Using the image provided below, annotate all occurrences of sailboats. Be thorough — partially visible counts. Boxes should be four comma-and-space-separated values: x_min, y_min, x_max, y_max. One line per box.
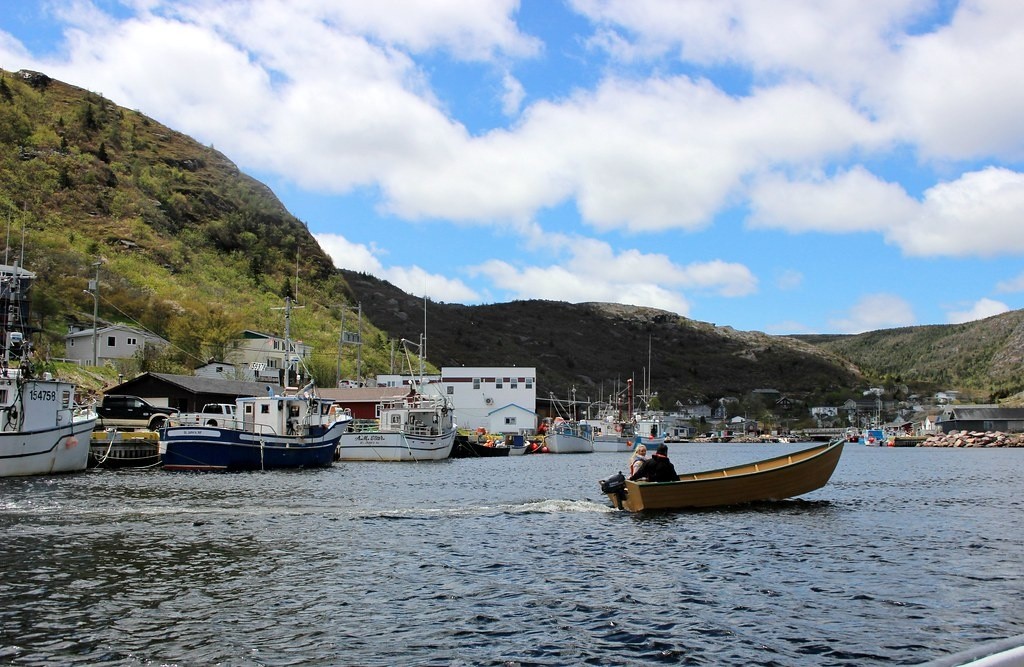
544, 332, 668, 453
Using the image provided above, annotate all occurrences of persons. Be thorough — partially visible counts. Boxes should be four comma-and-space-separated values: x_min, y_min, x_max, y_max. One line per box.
627, 443, 681, 482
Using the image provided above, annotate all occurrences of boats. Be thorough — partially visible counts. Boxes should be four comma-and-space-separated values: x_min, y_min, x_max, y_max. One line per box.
0, 201, 459, 478
845, 389, 887, 446
499, 440, 530, 456
466, 440, 511, 456
599, 437, 846, 513
